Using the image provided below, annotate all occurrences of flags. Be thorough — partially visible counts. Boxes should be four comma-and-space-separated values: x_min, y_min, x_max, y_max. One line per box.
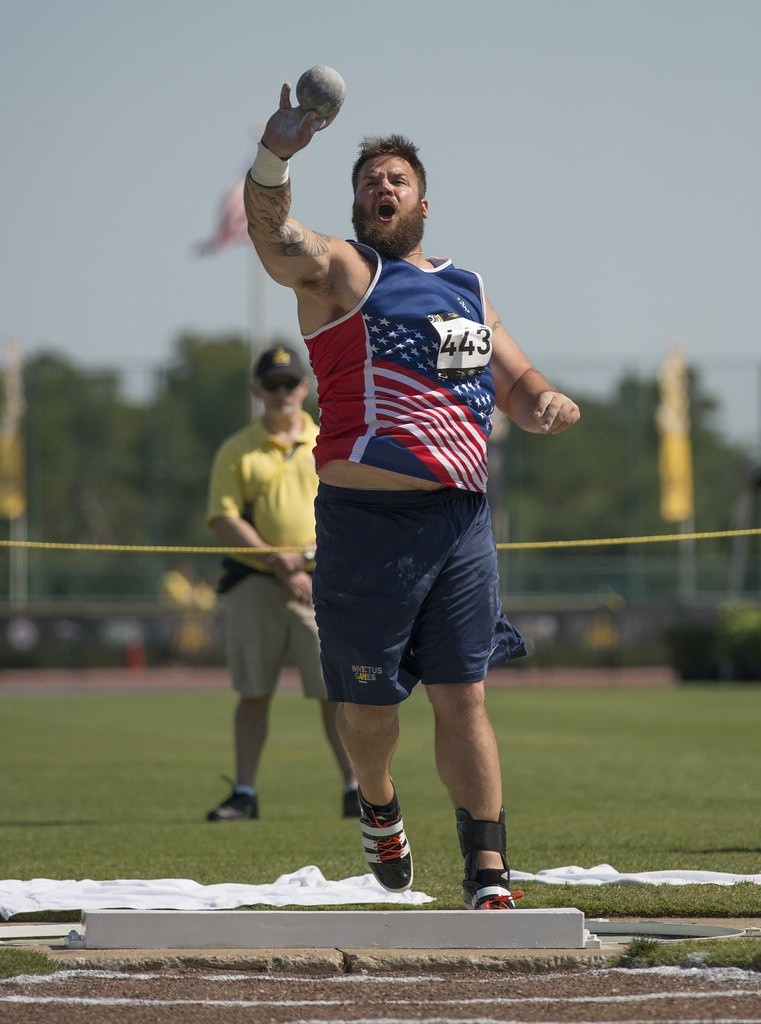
192, 181, 256, 255
0, 358, 30, 520
652, 344, 694, 523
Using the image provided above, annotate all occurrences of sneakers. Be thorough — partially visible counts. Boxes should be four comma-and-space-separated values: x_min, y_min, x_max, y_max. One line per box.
356, 775, 415, 894
343, 788, 362, 819
205, 773, 260, 823
460, 877, 525, 910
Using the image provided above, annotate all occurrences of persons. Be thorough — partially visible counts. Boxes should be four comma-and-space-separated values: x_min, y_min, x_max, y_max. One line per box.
245, 82, 579, 911
163, 346, 358, 824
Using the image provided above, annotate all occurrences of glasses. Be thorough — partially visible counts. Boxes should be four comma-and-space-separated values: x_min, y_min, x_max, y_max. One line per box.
264, 376, 300, 392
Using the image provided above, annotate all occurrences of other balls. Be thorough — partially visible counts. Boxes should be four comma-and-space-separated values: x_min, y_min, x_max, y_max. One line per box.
293, 64, 351, 119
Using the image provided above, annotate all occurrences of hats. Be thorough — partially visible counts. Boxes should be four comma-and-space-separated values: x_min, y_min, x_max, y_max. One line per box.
253, 342, 307, 387
